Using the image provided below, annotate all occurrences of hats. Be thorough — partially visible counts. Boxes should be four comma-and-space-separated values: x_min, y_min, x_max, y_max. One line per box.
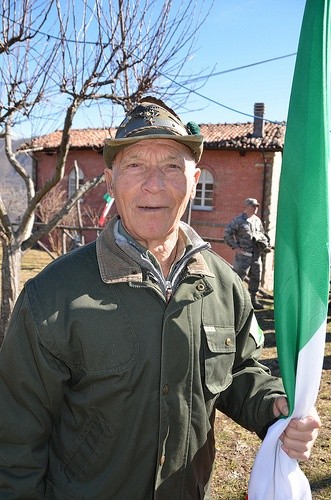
244, 198, 260, 207
102, 101, 204, 168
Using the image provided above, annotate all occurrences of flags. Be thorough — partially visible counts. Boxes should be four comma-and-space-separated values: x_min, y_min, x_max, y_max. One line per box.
246, 0, 327, 500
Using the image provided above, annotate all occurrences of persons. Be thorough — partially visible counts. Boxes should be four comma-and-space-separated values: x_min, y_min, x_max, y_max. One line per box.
221, 197, 273, 311
0, 97, 323, 500
